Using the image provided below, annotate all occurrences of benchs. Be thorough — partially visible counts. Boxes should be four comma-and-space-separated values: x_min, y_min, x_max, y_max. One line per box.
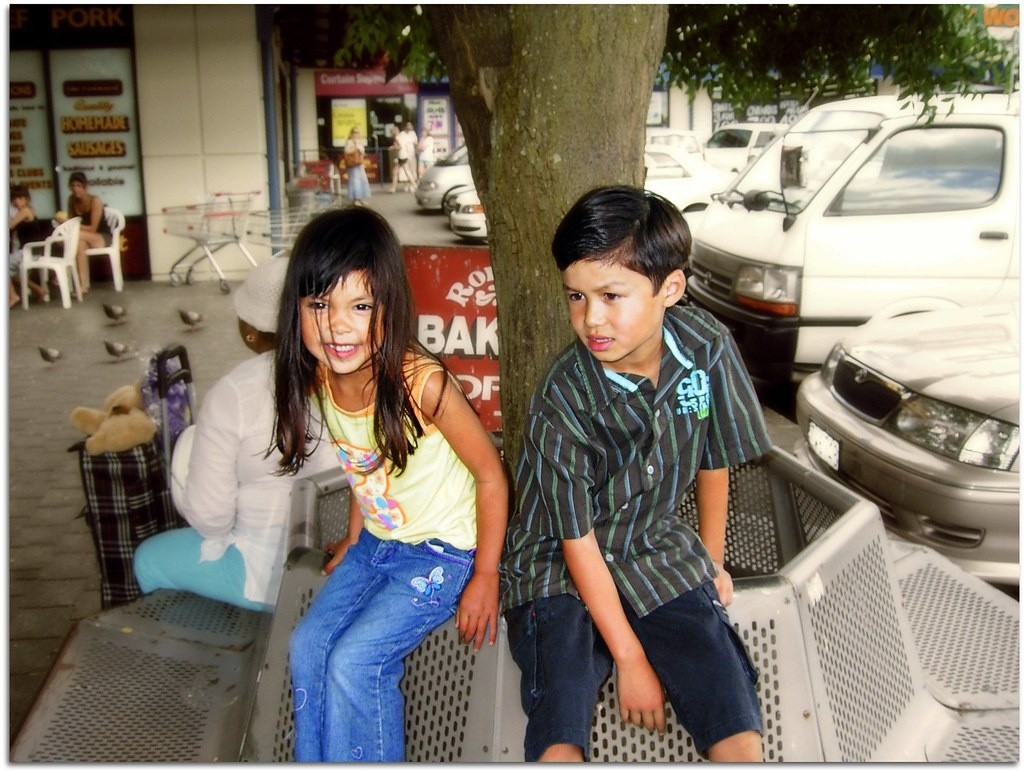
10, 449, 1019, 763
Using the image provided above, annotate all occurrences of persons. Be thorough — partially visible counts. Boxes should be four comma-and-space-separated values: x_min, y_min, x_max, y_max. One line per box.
264, 206, 509, 763
9, 184, 51, 310
387, 121, 435, 195
135, 256, 339, 612
344, 127, 372, 206
499, 184, 769, 763
53, 173, 114, 299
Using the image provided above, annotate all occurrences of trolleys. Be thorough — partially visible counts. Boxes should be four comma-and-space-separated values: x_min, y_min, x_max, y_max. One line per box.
246, 189, 357, 259
162, 191, 262, 294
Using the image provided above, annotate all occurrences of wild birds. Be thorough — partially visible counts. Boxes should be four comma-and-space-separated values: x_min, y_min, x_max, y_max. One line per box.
98, 301, 129, 326
173, 306, 206, 331
102, 339, 130, 362
36, 345, 67, 366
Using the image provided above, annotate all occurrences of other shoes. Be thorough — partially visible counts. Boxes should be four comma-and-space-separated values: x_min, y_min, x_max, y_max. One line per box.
38, 292, 50, 302
349, 200, 369, 208
47, 279, 61, 289
7, 296, 21, 309
70, 288, 90, 299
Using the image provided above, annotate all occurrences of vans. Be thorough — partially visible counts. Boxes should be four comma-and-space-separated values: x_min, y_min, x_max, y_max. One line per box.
690, 93, 1022, 397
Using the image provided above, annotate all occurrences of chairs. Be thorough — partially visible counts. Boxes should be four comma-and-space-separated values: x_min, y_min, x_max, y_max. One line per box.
79, 208, 127, 292
20, 217, 81, 311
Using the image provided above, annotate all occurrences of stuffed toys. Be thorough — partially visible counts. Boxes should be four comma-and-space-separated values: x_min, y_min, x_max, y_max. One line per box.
72, 384, 156, 456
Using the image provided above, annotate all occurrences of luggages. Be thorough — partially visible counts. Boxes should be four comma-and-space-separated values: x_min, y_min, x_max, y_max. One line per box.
69, 341, 197, 608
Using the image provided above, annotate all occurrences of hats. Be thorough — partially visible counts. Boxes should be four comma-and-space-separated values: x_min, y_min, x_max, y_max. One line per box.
69, 172, 87, 183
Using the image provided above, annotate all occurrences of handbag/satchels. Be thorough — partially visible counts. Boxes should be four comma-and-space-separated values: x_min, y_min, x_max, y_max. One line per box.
344, 149, 365, 169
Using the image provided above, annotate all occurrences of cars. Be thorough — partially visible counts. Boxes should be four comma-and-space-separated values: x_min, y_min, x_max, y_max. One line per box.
451, 143, 740, 247
794, 291, 1020, 585
699, 121, 790, 175
415, 125, 700, 217
